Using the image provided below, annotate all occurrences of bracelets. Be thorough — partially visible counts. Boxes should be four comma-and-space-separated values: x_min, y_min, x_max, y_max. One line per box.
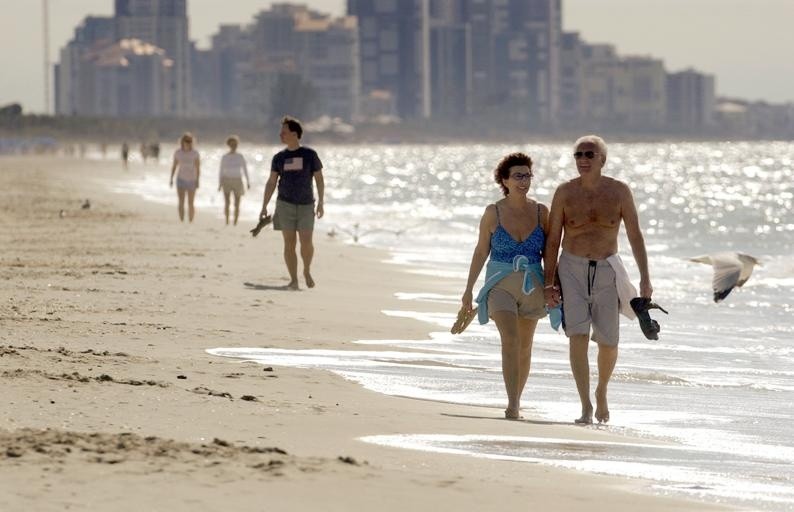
543, 285, 553, 289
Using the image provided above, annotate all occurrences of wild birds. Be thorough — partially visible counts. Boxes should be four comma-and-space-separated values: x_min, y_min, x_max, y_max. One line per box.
684, 252, 763, 304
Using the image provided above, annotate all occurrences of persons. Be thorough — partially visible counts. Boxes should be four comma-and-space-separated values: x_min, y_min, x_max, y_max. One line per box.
169, 132, 200, 223
259, 115, 325, 290
461, 152, 560, 418
543, 135, 652, 424
217, 135, 251, 226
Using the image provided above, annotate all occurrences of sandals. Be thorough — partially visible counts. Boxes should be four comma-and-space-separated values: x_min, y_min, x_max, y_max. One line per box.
629, 296, 661, 341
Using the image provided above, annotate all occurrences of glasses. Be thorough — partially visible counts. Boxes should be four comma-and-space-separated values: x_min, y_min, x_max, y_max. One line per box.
573, 152, 600, 160
509, 172, 531, 181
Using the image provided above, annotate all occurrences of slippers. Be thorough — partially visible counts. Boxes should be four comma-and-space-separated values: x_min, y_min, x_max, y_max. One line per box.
451, 304, 479, 334
250, 215, 271, 238
